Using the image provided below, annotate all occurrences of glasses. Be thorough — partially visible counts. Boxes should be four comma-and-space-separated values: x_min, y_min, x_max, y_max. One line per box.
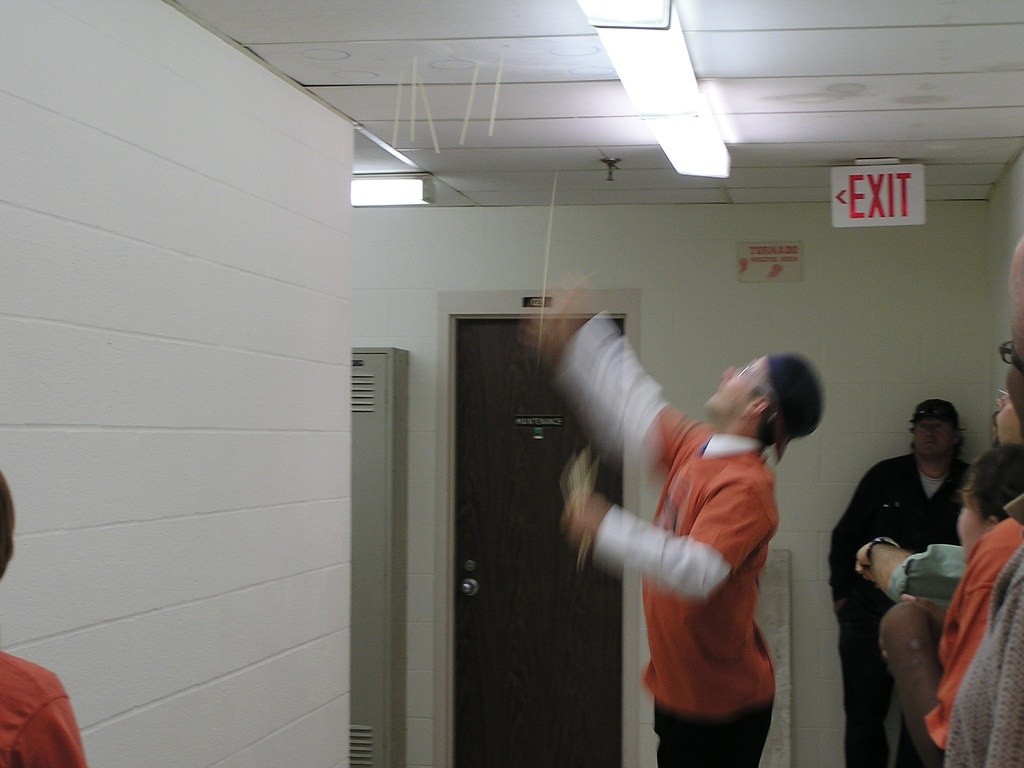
997, 388, 1010, 400
737, 363, 774, 397
1000, 339, 1017, 364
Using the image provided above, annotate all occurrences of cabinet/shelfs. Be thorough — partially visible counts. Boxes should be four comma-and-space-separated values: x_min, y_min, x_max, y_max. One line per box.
349, 347, 409, 768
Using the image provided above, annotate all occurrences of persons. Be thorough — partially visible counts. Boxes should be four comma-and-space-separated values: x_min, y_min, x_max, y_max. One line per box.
0, 469, 88, 768
556, 309, 825, 768
829, 233, 1023, 767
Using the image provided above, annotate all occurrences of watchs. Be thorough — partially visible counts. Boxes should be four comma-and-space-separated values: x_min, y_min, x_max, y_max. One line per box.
866, 537, 898, 564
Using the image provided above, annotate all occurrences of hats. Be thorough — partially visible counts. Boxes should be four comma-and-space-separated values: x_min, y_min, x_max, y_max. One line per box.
909, 399, 960, 430
768, 352, 822, 463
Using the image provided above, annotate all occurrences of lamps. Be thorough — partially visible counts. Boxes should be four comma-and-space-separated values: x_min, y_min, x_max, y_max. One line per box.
578, 0, 730, 178
351, 173, 436, 207
